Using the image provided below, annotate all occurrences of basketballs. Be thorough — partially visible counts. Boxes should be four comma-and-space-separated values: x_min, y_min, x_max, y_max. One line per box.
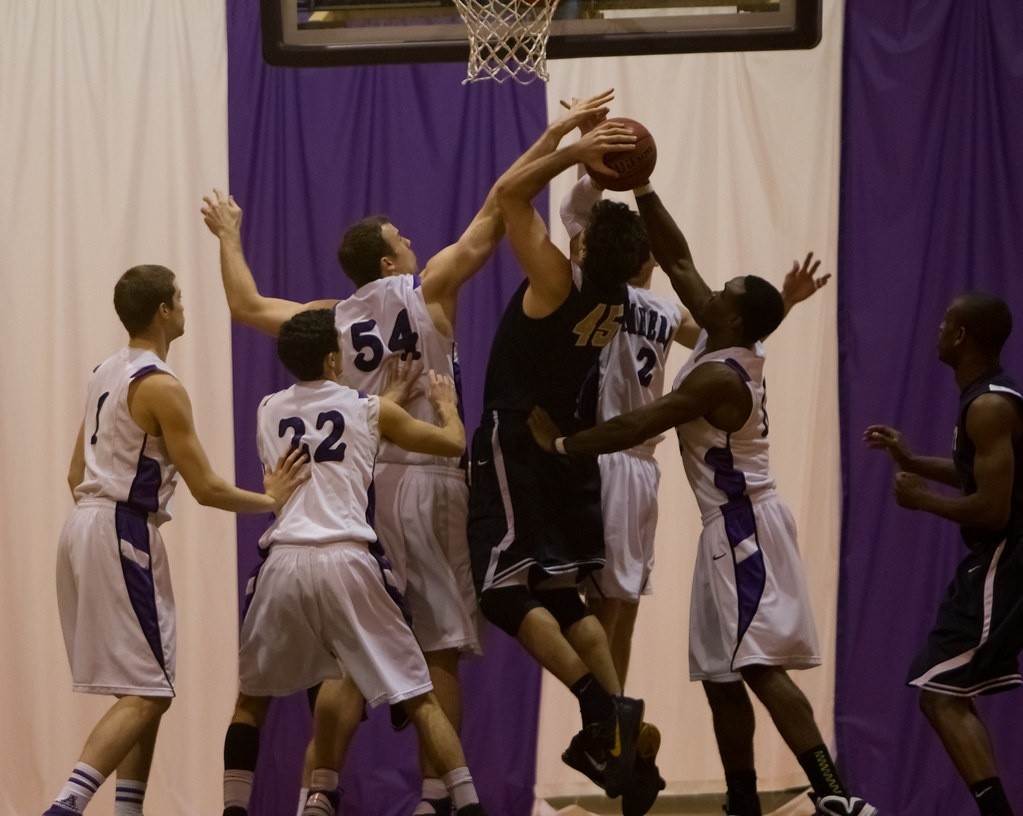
585, 117, 658, 192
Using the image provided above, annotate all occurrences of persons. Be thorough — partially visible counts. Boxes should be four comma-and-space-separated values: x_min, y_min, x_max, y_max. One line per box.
199, 88, 878, 816
862, 293, 1023, 816
41, 265, 313, 816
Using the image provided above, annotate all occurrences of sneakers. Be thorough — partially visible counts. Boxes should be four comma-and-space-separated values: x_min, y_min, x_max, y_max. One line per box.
412, 795, 458, 816
302, 787, 340, 816
561, 696, 666, 816
806, 790, 879, 816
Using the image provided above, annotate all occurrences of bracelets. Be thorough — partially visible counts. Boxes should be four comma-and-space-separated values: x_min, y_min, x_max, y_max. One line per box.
554, 436, 568, 455
633, 182, 654, 197
